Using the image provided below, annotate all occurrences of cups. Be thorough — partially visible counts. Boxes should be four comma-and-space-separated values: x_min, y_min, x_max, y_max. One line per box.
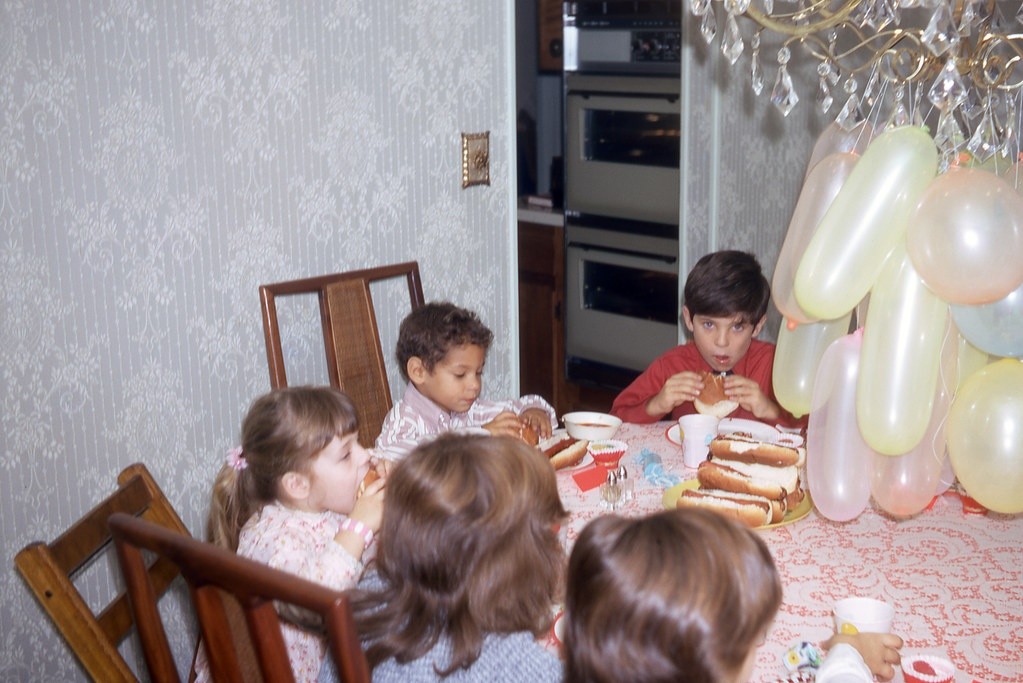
677, 413, 720, 468
831, 596, 895, 636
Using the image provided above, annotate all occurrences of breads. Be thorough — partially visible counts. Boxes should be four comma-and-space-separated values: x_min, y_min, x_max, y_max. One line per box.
358, 466, 386, 498
692, 370, 740, 418
508, 417, 540, 447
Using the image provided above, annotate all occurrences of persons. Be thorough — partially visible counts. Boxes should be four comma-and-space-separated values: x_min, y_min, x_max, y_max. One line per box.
193, 388, 396, 683
317, 434, 564, 683
611, 250, 809, 432
375, 303, 558, 447
562, 505, 903, 683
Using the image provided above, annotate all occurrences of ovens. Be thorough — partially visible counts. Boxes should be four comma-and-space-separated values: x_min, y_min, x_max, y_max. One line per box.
563, 69, 681, 373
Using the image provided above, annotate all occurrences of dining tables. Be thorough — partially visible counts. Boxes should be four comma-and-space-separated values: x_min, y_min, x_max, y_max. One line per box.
530, 418, 1023, 683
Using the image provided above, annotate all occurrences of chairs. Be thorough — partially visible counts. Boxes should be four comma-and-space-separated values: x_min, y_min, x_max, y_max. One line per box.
14, 461, 203, 683
107, 511, 373, 683
258, 261, 428, 449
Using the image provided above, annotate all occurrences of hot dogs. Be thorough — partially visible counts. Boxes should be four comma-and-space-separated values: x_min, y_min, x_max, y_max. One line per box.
676, 430, 804, 526
532, 433, 591, 470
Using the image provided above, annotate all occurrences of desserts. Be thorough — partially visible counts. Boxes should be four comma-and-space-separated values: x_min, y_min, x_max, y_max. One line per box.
909, 659, 938, 677
591, 443, 618, 453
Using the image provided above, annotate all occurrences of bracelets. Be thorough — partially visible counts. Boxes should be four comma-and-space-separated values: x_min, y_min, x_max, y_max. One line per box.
339, 518, 373, 550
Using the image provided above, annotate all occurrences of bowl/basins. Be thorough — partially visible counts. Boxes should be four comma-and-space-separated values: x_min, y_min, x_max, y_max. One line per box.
563, 411, 623, 441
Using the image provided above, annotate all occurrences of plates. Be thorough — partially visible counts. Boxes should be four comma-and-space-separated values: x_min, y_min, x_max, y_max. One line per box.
661, 478, 814, 530
665, 418, 783, 449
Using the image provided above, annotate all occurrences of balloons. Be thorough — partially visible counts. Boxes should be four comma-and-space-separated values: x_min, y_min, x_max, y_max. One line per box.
772, 121, 1023, 522
